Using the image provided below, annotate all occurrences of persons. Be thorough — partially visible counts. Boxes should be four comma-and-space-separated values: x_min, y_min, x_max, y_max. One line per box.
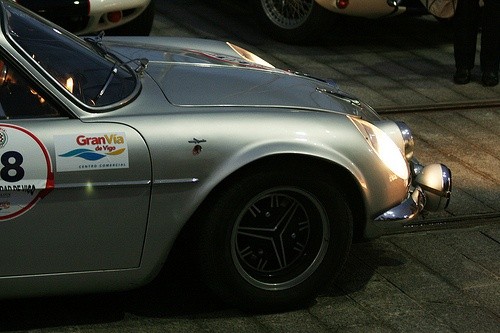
452, 0, 500, 87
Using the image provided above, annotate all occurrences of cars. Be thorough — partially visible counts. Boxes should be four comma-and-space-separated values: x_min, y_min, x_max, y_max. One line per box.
0, 0, 452, 314
9, 0, 156, 36
237, 0, 457, 44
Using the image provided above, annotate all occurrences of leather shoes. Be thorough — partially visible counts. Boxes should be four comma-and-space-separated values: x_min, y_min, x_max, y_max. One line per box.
482, 71, 498, 87
454, 68, 470, 84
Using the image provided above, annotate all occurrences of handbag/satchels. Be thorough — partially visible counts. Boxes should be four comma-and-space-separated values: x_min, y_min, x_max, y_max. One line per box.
421, 0, 457, 18
